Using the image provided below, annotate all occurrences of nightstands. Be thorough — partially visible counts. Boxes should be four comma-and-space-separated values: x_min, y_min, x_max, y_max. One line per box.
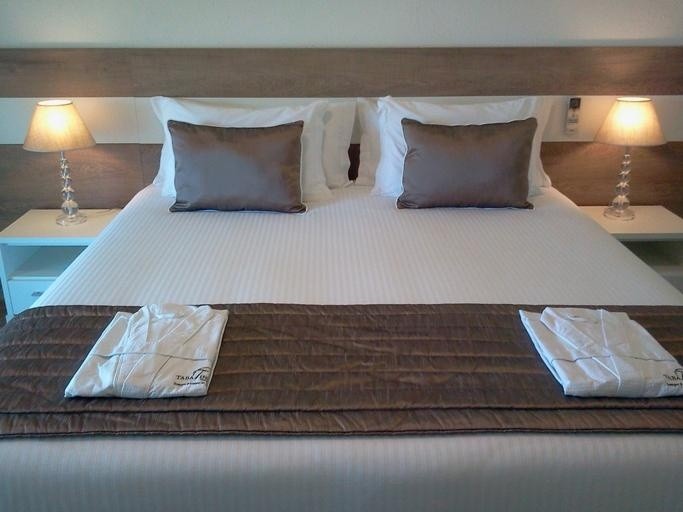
0, 207, 127, 323
575, 204, 681, 294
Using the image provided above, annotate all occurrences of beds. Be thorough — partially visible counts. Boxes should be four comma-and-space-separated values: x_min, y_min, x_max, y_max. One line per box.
0, 181, 683, 511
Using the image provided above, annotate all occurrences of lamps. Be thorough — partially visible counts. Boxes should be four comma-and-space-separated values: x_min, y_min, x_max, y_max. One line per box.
19, 96, 100, 227
592, 93, 671, 223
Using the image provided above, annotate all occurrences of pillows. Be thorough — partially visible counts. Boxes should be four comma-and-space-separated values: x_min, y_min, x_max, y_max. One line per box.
396, 118, 538, 211
159, 96, 334, 204
150, 97, 357, 189
166, 119, 308, 214
352, 95, 555, 188
369, 95, 547, 198
